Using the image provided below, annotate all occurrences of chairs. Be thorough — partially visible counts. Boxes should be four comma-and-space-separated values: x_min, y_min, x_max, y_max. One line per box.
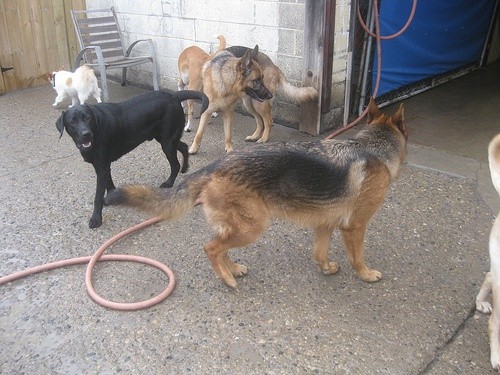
70, 6, 159, 103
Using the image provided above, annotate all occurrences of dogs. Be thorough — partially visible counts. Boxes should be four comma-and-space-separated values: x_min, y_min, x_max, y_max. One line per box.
55, 89, 209, 229
101, 96, 408, 291
178, 35, 318, 155
46, 62, 102, 109
475, 133, 499, 373
